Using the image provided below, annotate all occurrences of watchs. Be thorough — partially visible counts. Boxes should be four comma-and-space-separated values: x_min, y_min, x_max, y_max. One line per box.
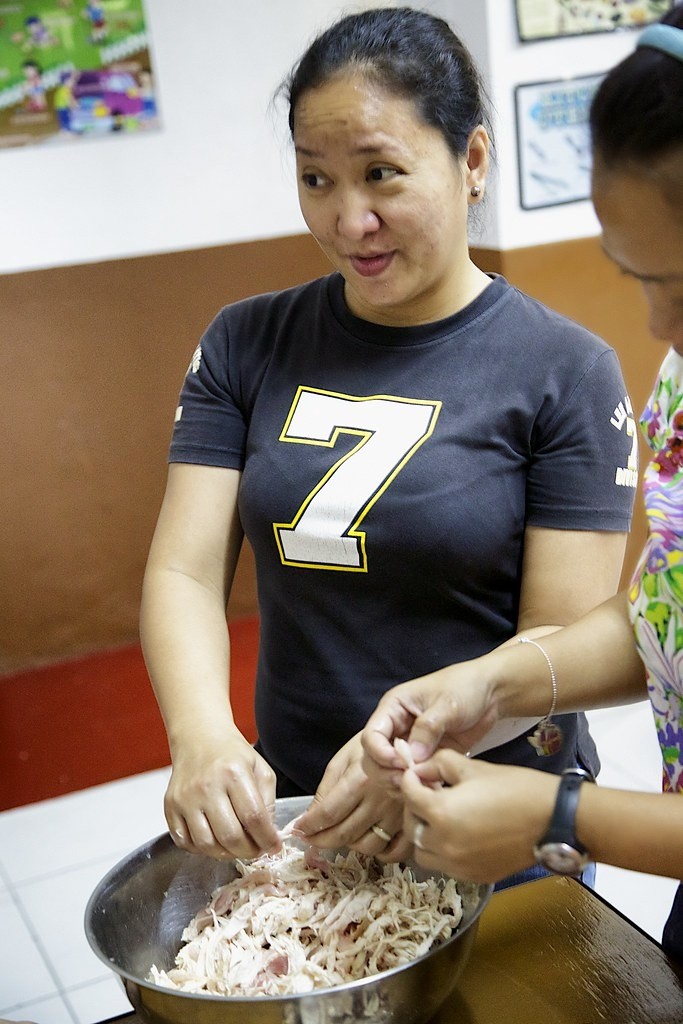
534, 768, 599, 878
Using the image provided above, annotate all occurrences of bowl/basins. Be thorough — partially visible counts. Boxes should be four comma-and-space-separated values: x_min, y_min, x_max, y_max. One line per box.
84, 795, 495, 1024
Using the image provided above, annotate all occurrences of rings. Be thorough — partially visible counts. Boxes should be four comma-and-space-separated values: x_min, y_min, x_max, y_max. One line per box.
415, 822, 423, 848
370, 826, 392, 843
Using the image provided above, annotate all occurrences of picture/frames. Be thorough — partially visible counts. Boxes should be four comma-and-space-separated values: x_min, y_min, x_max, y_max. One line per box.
512, 0, 675, 43
514, 69, 615, 212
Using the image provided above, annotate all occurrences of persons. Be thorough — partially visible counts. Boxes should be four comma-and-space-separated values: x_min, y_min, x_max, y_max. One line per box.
137, 3, 645, 894
360, 0, 683, 968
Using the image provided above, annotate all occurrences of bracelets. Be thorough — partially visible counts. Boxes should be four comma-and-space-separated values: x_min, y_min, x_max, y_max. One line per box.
517, 637, 557, 725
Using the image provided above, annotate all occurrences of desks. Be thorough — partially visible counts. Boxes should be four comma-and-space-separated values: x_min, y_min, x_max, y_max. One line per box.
89, 870, 683, 1024
